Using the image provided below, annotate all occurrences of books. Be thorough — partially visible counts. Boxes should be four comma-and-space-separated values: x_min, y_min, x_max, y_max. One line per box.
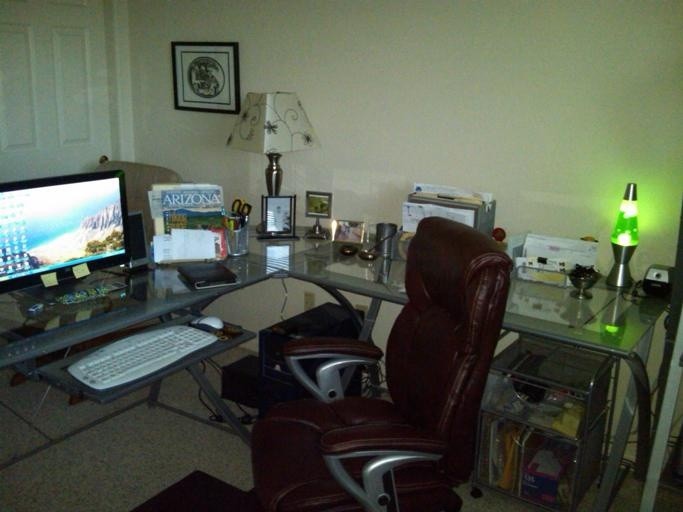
177, 262, 240, 289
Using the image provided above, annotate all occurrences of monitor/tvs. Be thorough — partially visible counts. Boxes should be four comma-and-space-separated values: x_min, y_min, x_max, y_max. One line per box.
0, 168, 133, 301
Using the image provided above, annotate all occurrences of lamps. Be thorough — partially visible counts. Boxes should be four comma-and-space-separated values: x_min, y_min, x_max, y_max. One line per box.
226, 91, 319, 234
605, 182, 642, 287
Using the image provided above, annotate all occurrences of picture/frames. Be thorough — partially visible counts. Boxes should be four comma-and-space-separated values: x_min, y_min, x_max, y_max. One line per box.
170, 41, 241, 114
305, 190, 332, 217
331, 219, 365, 245
256, 195, 299, 242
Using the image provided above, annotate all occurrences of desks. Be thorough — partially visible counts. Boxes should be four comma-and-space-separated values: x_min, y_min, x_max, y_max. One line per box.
225, 222, 674, 512
0, 252, 281, 450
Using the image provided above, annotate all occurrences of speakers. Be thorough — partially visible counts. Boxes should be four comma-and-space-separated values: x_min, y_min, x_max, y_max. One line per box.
127, 209, 152, 268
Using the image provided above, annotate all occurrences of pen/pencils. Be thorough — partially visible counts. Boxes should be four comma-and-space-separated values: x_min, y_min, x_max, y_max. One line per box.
224, 214, 248, 253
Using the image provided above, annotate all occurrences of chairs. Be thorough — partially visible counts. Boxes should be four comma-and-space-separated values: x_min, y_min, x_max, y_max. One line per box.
10, 155, 179, 403
250, 212, 511, 512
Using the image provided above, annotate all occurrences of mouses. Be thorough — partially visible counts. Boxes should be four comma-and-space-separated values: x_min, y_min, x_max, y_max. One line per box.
190, 315, 225, 331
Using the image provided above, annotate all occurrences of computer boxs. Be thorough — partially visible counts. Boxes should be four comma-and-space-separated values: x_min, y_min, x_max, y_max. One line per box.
259, 302, 365, 414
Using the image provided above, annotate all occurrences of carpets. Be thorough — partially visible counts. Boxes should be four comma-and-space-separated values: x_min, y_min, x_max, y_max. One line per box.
128, 470, 258, 512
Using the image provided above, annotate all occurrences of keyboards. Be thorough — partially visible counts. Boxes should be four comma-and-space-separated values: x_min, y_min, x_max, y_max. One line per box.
67, 325, 219, 391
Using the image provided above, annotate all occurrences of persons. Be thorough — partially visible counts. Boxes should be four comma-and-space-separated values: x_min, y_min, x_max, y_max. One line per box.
274, 206, 285, 231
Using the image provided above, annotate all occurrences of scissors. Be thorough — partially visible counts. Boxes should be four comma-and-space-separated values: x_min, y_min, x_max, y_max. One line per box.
231, 198, 253, 216
